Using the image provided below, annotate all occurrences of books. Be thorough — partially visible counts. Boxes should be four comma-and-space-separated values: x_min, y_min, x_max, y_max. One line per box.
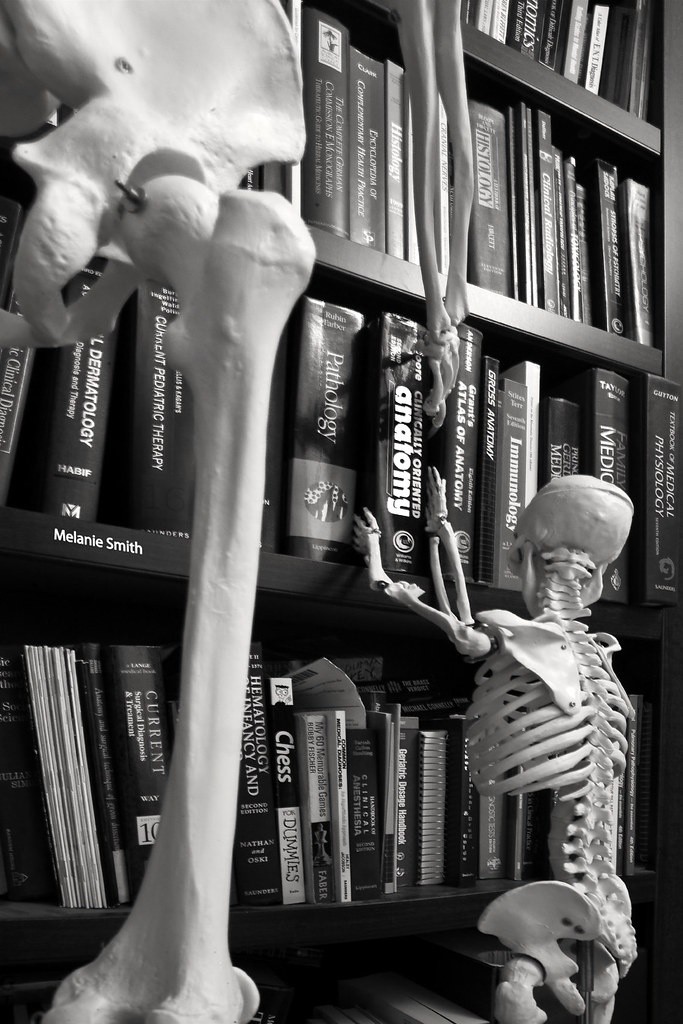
0, 194, 683, 606
56, 0, 654, 347
0, 643, 658, 1023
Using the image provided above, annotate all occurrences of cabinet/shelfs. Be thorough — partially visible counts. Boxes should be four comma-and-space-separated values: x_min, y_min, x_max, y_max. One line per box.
0, 0, 683, 1024
